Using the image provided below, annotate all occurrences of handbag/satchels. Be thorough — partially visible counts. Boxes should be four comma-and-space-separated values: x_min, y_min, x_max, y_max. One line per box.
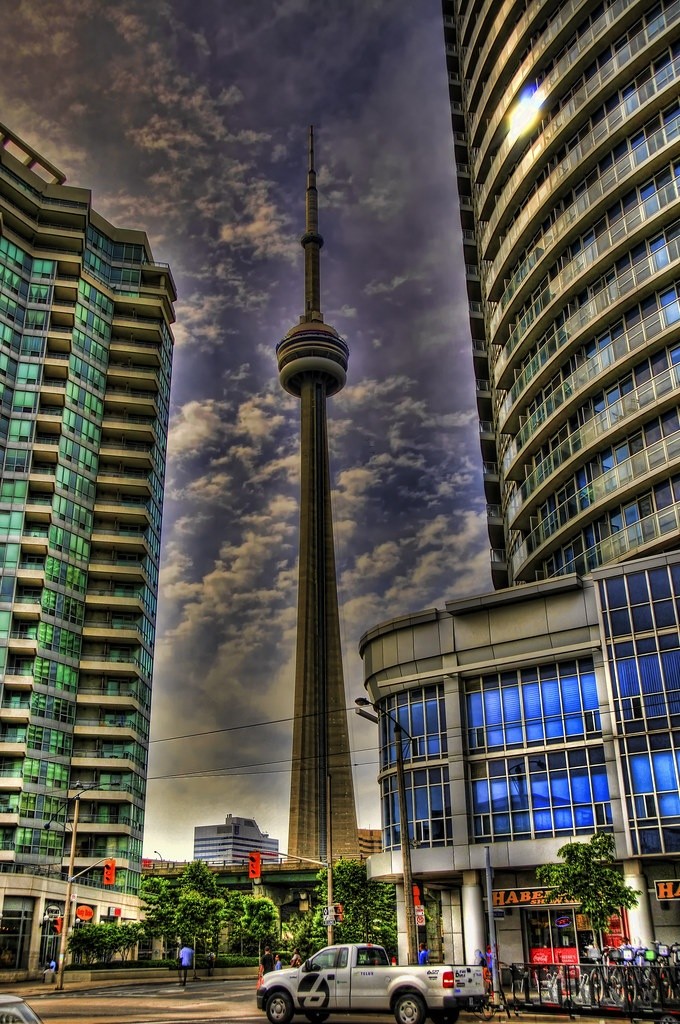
290, 954, 301, 967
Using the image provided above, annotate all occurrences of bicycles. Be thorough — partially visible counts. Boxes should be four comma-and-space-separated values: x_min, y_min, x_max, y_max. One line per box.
588, 938, 680, 1008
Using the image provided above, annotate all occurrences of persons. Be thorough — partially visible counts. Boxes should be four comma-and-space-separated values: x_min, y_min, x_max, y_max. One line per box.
275, 953, 282, 970
176, 940, 196, 987
206, 949, 216, 978
258, 946, 273, 980
290, 948, 302, 967
418, 943, 430, 965
43, 958, 57, 983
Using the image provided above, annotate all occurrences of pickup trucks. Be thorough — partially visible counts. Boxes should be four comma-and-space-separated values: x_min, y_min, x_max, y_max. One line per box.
253, 942, 490, 1024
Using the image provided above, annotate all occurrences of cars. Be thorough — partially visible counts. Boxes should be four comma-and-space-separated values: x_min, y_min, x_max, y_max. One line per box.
0, 993, 45, 1024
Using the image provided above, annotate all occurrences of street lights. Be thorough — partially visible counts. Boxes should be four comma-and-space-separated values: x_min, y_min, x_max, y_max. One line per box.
153, 850, 163, 867
44, 780, 120, 991
508, 759, 546, 832
354, 697, 418, 964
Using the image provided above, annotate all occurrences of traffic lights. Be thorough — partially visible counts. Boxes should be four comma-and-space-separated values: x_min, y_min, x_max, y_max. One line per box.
52, 918, 61, 933
104, 858, 116, 885
249, 851, 261, 879
334, 904, 344, 922
